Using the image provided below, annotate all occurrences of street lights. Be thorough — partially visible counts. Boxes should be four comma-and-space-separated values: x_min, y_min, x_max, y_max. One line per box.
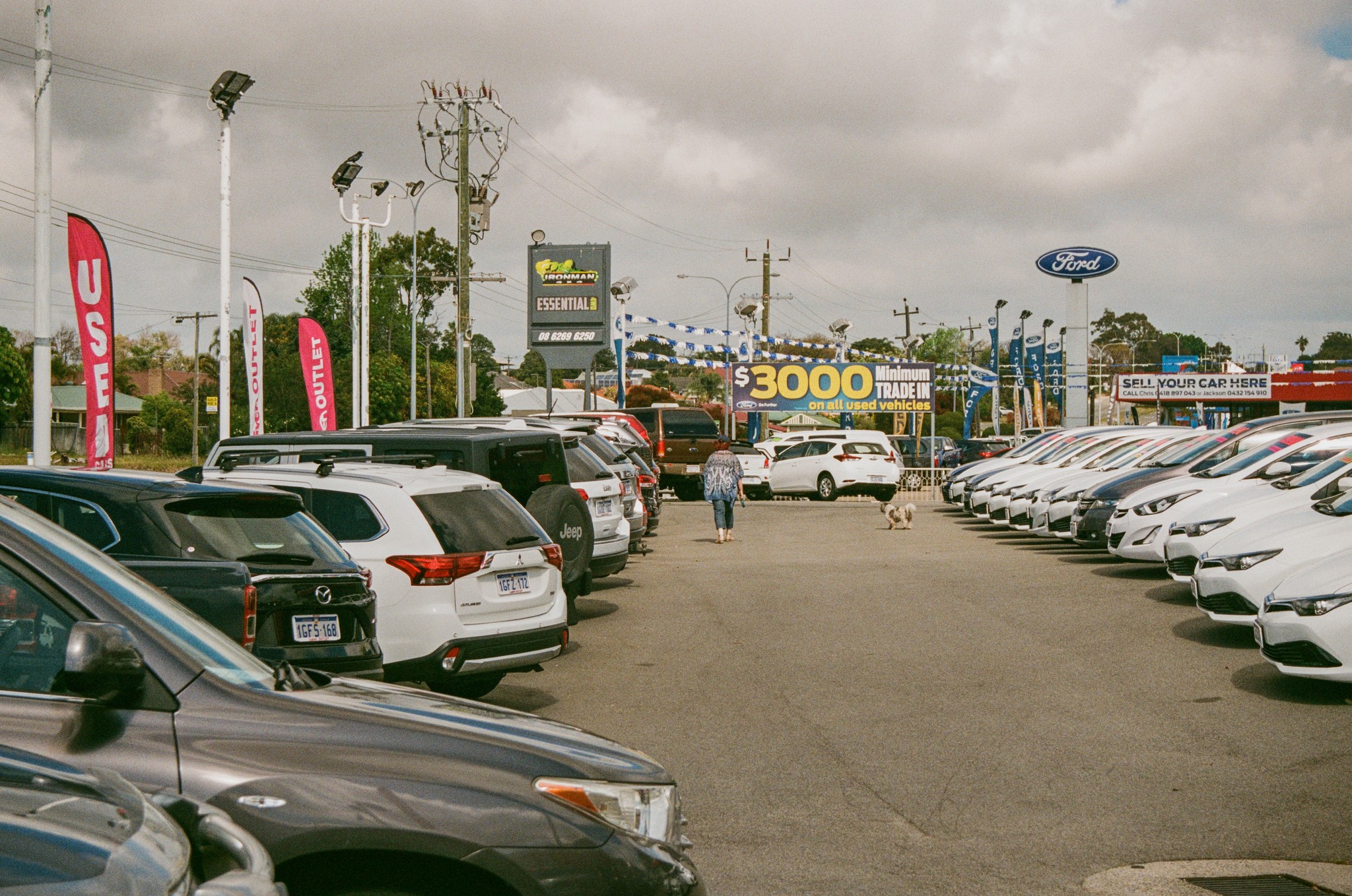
992, 298, 1009, 435
1058, 326, 1066, 425
826, 316, 855, 431
1018, 309, 1033, 430
610, 275, 640, 411
325, 149, 425, 431
897, 331, 925, 435
206, 69, 259, 438
734, 296, 758, 444
1040, 317, 1055, 426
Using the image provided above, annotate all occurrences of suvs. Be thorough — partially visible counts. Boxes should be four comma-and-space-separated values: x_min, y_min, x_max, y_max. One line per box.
481, 413, 664, 556
0, 458, 385, 685
342, 419, 633, 577
606, 406, 728, 501
0, 493, 697, 896
153, 456, 574, 702
201, 426, 594, 625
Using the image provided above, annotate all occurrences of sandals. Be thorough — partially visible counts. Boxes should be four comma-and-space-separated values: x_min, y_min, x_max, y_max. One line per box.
715, 534, 725, 544
725, 532, 735, 542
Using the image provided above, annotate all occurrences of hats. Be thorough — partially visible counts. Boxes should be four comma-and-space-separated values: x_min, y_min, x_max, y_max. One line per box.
717, 434, 731, 443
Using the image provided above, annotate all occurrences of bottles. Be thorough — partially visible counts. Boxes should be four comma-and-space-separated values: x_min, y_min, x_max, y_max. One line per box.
740, 498, 745, 507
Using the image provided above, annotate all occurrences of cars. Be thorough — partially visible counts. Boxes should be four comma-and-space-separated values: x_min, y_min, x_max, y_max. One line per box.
723, 422, 1226, 562
1189, 477, 1352, 627
1163, 445, 1351, 583
2, 733, 291, 896
1253, 546, 1352, 684
1067, 407, 1352, 548
1104, 422, 1352, 562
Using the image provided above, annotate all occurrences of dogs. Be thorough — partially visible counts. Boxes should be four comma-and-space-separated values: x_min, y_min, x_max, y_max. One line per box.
880, 501, 916, 530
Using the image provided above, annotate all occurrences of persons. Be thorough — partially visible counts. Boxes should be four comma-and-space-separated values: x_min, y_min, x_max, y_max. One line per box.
701, 434, 745, 543
